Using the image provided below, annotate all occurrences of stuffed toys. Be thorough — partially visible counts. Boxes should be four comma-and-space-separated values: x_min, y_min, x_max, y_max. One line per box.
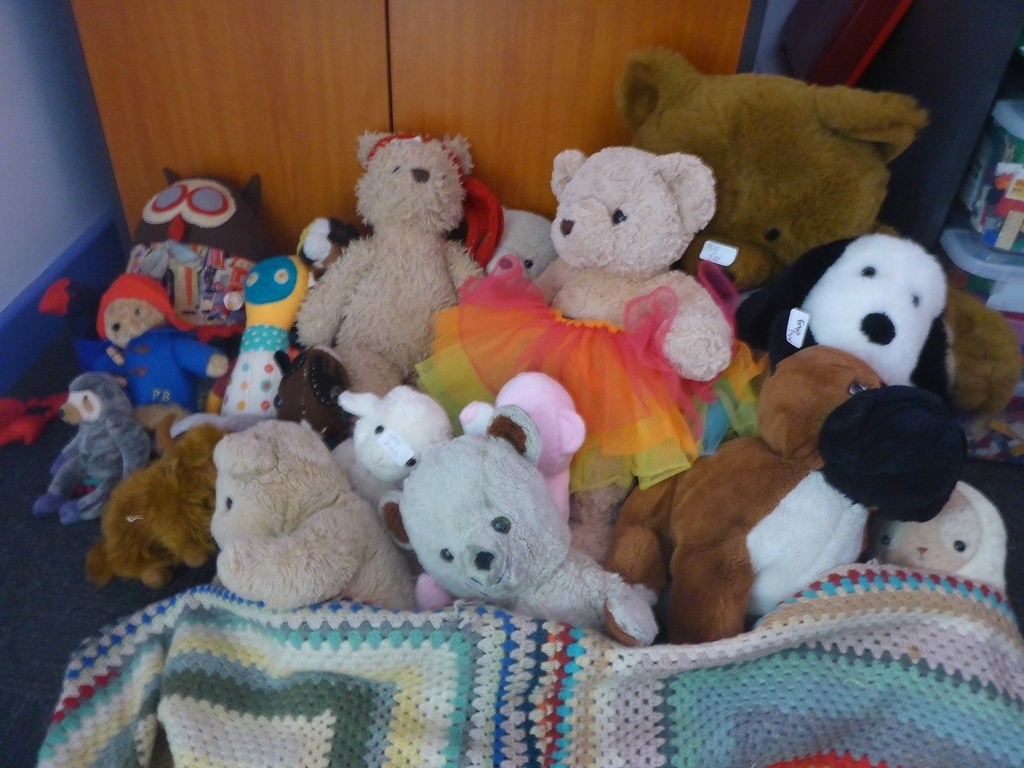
528, 145, 735, 563
607, 344, 965, 647
735, 231, 947, 398
484, 209, 559, 292
614, 45, 1022, 418
330, 383, 454, 506
210, 420, 413, 611
865, 480, 1008, 597
207, 254, 310, 430
292, 130, 488, 396
96, 274, 229, 432
32, 372, 152, 525
0, 167, 266, 446
85, 425, 232, 591
272, 348, 350, 453
415, 372, 585, 613
378, 405, 658, 647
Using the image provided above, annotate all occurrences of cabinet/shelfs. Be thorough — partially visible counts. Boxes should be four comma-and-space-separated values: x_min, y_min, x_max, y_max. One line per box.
854, 0, 1024, 253
71, 1, 751, 267
